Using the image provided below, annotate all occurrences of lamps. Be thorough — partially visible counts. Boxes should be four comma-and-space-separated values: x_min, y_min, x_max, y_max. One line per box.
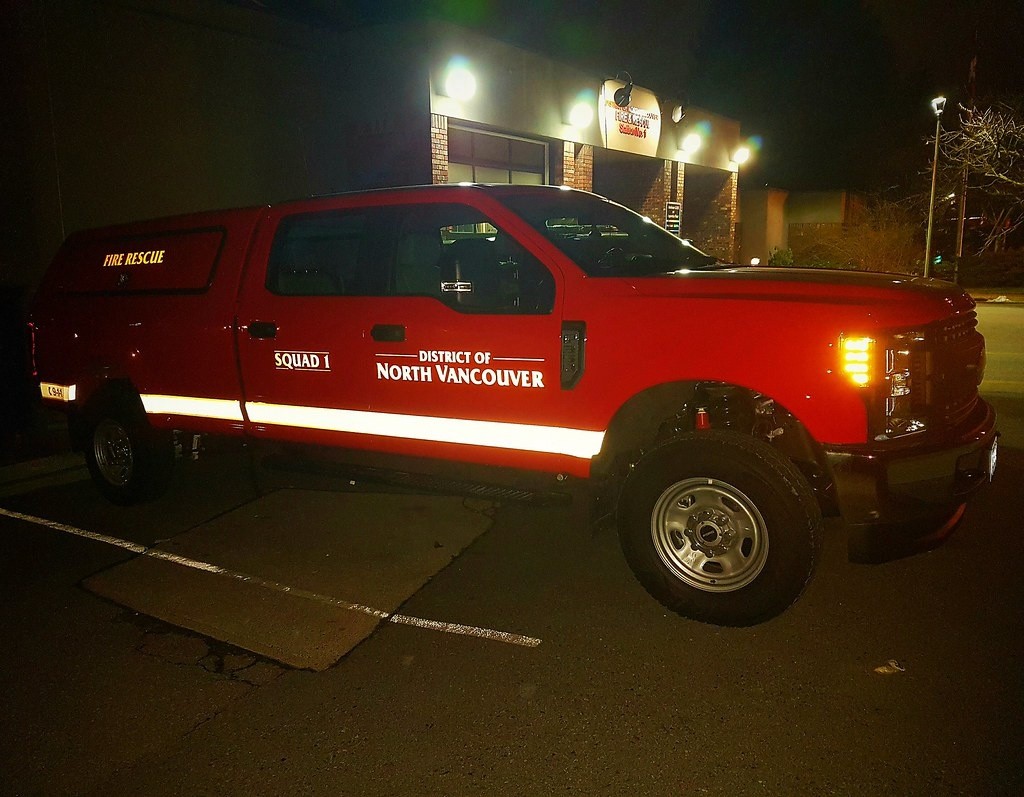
658, 93, 689, 124
602, 71, 634, 108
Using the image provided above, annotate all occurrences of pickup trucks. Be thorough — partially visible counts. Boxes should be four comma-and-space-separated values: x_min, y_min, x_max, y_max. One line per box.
27, 184, 1000, 627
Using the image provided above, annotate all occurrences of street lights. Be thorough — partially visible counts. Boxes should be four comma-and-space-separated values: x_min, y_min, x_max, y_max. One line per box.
924, 95, 947, 277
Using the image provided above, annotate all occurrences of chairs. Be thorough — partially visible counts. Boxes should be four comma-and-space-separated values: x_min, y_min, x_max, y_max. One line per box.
495, 230, 522, 303
279, 240, 334, 297
394, 232, 443, 298
